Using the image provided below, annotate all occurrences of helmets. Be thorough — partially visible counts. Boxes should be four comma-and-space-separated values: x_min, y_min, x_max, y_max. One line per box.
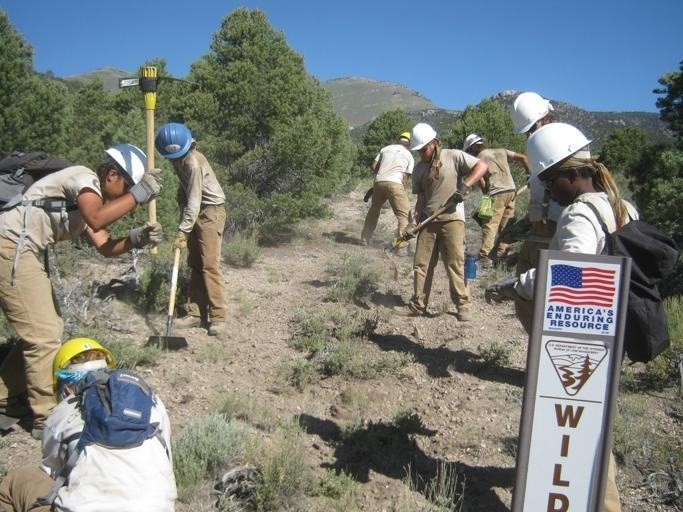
409, 123, 438, 151
52, 337, 116, 392
511, 92, 549, 135
104, 143, 146, 184
399, 132, 410, 139
526, 122, 593, 186
463, 134, 484, 151
155, 123, 193, 159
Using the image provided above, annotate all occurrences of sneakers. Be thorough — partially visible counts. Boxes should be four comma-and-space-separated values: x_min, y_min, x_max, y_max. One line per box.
362, 236, 370, 246
457, 298, 474, 321
393, 303, 426, 316
176, 314, 206, 329
207, 320, 226, 335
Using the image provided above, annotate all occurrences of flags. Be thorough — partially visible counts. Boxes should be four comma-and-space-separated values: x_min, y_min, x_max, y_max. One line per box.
551, 259, 616, 309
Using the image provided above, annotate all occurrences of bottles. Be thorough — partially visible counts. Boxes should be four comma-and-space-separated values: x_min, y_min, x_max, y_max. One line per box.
466, 254, 477, 279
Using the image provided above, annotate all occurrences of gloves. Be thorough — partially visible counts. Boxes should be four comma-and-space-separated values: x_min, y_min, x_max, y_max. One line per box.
130, 169, 164, 205
485, 278, 520, 305
402, 223, 417, 241
173, 229, 189, 249
128, 220, 163, 249
453, 184, 469, 203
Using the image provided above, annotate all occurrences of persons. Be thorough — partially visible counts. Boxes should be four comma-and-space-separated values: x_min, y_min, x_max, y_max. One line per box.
359, 129, 415, 257
0, 140, 164, 439
477, 120, 641, 510
153, 123, 228, 336
401, 122, 486, 323
491, 91, 569, 343
462, 132, 531, 260
0, 335, 181, 511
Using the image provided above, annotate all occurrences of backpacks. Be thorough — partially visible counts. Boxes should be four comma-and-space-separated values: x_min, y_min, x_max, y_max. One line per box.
76, 370, 153, 449
0, 149, 79, 213
575, 193, 682, 366
